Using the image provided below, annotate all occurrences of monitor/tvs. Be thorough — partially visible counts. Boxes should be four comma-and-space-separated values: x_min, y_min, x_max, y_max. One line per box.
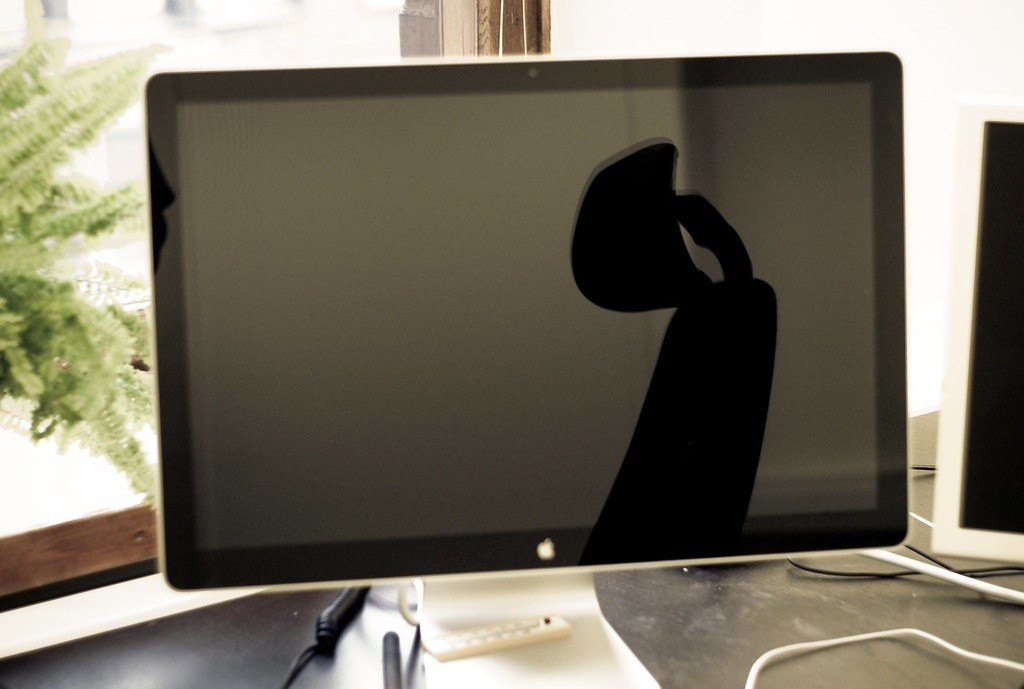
142, 53, 912, 689
932, 103, 1024, 564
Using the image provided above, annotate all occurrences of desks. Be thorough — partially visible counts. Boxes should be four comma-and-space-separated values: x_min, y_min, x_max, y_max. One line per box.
0, 406, 1024, 689
